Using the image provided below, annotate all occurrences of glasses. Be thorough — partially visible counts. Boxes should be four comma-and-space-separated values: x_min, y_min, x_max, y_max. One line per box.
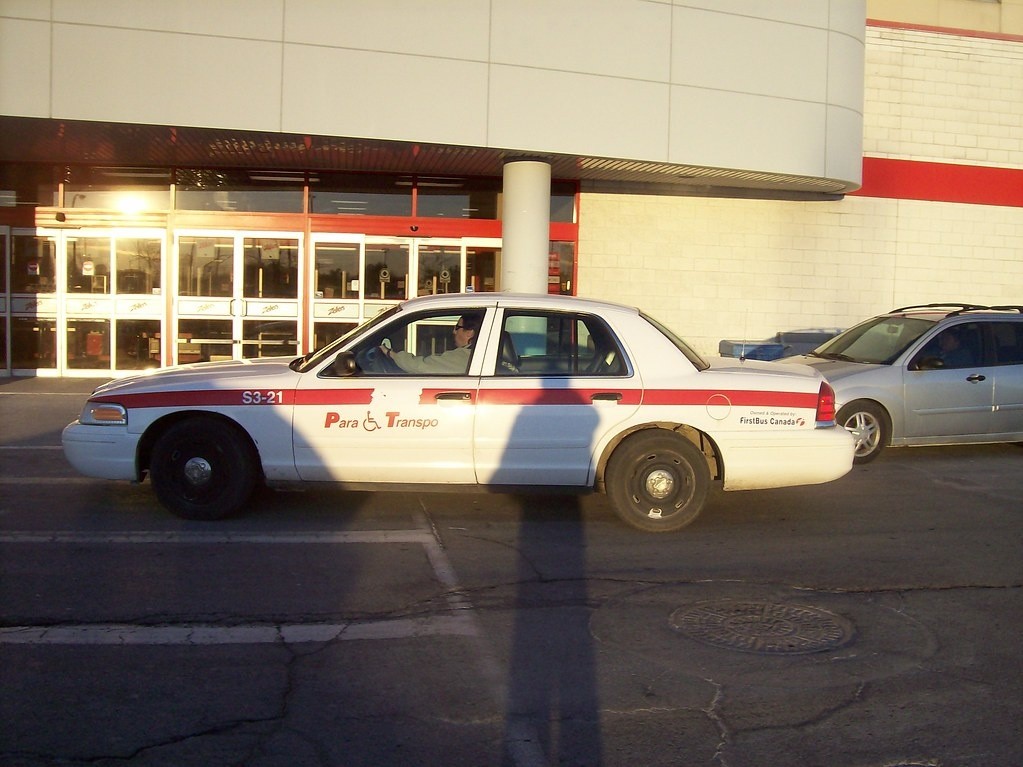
455, 323, 464, 330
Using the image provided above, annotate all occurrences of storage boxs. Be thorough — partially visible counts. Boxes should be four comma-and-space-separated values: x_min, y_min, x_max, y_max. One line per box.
548, 253, 562, 295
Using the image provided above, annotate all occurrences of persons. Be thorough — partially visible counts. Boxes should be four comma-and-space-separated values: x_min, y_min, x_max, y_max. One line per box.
934, 325, 975, 368
378, 312, 481, 376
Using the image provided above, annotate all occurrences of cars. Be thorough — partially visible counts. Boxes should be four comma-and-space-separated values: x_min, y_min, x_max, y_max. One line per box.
61, 293, 858, 533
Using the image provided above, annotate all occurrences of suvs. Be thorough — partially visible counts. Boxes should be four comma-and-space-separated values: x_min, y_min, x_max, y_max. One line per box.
770, 302, 1023, 467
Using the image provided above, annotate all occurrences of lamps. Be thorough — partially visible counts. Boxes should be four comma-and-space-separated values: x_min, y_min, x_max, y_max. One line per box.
566, 280, 571, 291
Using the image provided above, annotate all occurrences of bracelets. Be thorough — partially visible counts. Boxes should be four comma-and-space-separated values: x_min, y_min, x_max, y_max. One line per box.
387, 349, 392, 359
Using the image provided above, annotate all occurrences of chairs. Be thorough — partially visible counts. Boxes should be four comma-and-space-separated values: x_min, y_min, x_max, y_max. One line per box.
582, 351, 620, 374
496, 331, 521, 375
